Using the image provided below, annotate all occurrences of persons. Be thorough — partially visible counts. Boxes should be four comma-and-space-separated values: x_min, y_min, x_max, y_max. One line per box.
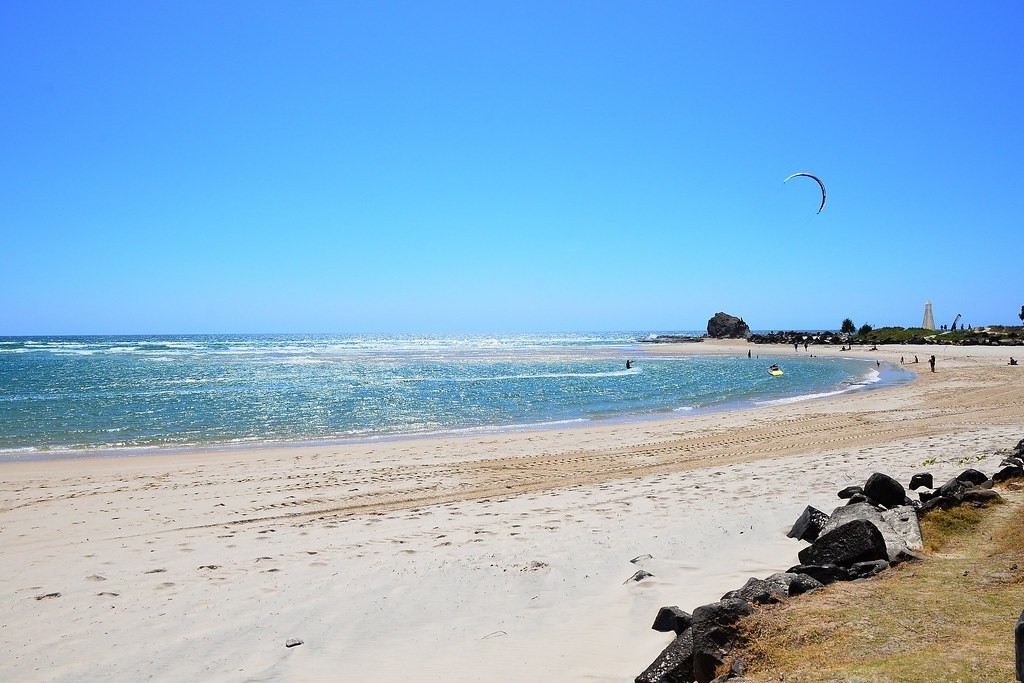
842, 345, 851, 351
748, 350, 751, 357
929, 355, 935, 373
941, 325, 947, 331
915, 356, 918, 363
901, 356, 904, 364
794, 340, 798, 349
877, 361, 880, 367
805, 339, 808, 351
1010, 357, 1014, 365
961, 324, 964, 330
626, 360, 633, 369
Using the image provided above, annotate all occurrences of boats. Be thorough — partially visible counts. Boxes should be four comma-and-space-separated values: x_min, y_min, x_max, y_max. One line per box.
767, 366, 783, 377
